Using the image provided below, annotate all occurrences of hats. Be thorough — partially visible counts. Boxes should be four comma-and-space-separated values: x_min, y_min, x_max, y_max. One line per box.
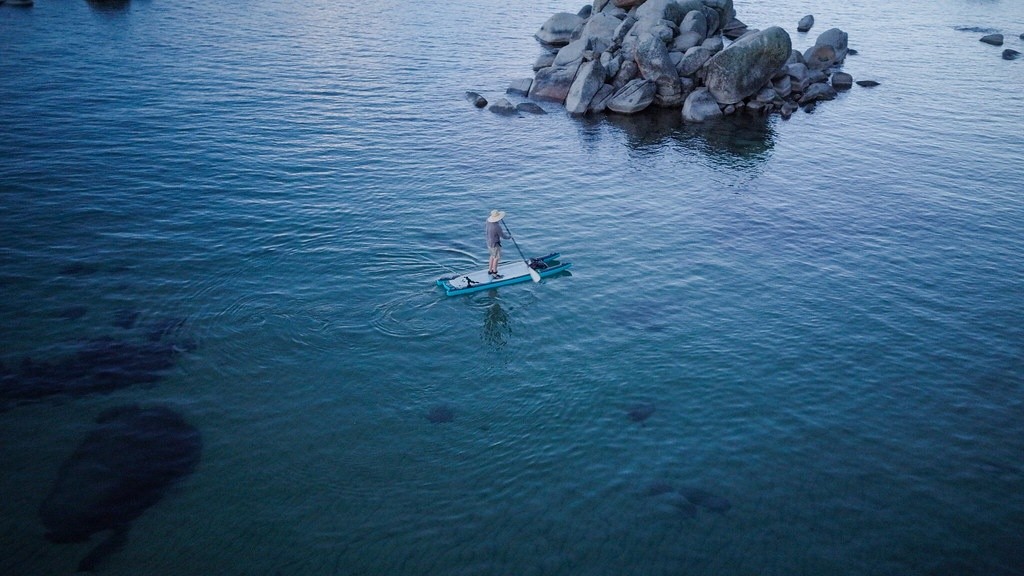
487, 210, 505, 223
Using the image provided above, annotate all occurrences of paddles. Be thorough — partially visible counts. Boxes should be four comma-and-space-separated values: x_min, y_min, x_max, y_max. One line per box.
501, 219, 542, 284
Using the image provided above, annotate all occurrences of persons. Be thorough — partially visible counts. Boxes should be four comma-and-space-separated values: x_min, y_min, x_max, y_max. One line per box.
486, 209, 513, 279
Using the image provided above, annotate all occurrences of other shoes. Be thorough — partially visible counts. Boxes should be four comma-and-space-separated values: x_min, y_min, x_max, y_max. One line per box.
489, 272, 503, 278
